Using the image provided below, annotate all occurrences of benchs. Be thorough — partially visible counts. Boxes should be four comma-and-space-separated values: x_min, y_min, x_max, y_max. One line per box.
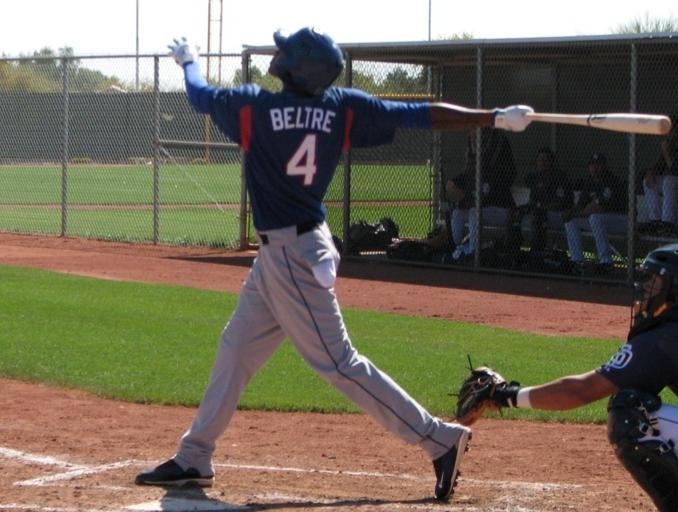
436, 186, 678, 273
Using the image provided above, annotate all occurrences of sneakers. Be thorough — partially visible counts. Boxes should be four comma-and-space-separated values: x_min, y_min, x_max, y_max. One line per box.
133, 454, 215, 489
430, 425, 472, 501
571, 260, 616, 274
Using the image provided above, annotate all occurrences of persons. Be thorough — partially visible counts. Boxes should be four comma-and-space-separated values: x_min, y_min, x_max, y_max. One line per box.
445, 125, 515, 263
505, 145, 575, 271
134, 22, 535, 506
451, 241, 678, 511
642, 130, 678, 229
561, 150, 632, 276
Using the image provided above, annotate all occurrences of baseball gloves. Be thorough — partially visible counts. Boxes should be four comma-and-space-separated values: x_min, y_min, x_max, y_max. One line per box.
449, 355, 517, 426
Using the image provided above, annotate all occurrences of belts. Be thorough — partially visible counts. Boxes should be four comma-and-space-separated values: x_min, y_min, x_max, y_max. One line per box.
255, 216, 322, 246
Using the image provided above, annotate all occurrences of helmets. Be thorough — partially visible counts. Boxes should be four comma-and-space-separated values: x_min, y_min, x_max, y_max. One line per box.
629, 242, 677, 331
271, 24, 345, 91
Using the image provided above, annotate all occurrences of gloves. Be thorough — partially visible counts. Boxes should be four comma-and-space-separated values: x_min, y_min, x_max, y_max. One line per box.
492, 103, 534, 133
164, 35, 202, 68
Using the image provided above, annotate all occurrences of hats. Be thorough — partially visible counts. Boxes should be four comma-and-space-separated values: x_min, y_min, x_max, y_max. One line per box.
586, 151, 607, 165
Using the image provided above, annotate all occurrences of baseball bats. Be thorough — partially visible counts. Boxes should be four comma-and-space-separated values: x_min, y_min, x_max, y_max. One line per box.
524, 111, 672, 136
